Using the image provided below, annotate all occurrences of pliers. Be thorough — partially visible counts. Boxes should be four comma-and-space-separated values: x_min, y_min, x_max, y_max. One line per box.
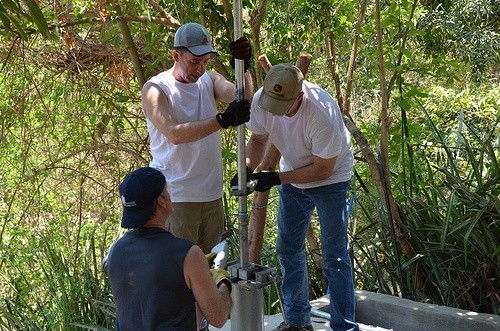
205, 230, 233, 271
229, 169, 272, 196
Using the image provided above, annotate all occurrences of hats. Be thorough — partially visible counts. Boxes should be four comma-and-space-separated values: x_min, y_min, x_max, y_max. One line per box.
258, 62, 304, 116
174, 22, 218, 57
119, 167, 165, 228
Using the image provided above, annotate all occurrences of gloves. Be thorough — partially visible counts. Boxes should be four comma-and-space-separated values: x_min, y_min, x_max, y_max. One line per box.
216, 99, 251, 129
230, 165, 281, 192
229, 37, 251, 73
205, 252, 232, 294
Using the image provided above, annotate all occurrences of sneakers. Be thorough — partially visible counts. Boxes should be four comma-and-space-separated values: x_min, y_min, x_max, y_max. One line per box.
271, 322, 314, 331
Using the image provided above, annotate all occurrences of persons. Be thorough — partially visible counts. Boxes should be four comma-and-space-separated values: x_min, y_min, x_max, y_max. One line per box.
230, 63, 361, 331
107, 167, 234, 331
140, 22, 252, 331
